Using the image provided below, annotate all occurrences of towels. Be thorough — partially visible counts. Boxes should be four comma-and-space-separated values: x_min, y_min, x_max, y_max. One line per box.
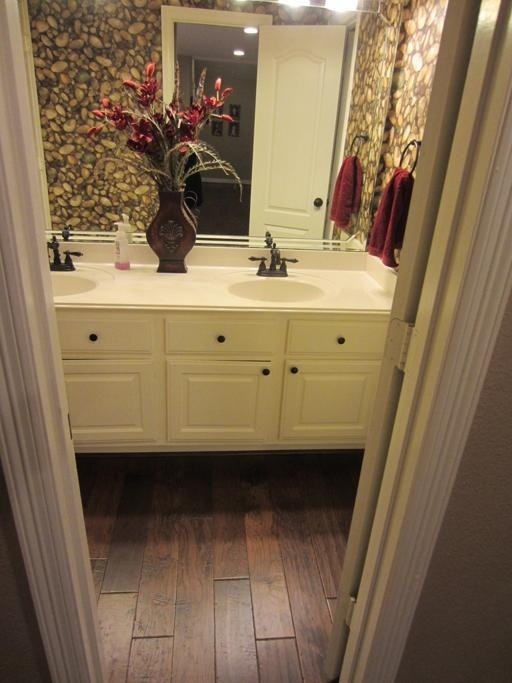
330, 157, 362, 229
368, 168, 414, 268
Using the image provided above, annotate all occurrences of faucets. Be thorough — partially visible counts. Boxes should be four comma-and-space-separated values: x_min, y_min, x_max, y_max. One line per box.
269, 242, 281, 270
264, 232, 273, 249
46, 236, 61, 265
62, 226, 70, 241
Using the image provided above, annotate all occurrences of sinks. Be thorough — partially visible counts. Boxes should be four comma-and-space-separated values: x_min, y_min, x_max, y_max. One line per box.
52, 271, 98, 298
230, 279, 323, 303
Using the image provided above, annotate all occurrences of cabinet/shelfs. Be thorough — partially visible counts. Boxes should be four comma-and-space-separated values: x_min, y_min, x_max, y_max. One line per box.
166, 317, 385, 444
59, 320, 156, 445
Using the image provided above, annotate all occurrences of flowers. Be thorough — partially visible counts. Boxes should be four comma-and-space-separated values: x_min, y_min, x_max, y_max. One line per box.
85, 58, 243, 201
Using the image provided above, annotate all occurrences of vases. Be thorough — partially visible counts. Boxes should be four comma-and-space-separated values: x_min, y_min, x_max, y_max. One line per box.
142, 191, 196, 272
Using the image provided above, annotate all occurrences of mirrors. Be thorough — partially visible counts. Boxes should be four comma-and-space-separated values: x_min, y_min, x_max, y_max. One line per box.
18, 1, 448, 250
